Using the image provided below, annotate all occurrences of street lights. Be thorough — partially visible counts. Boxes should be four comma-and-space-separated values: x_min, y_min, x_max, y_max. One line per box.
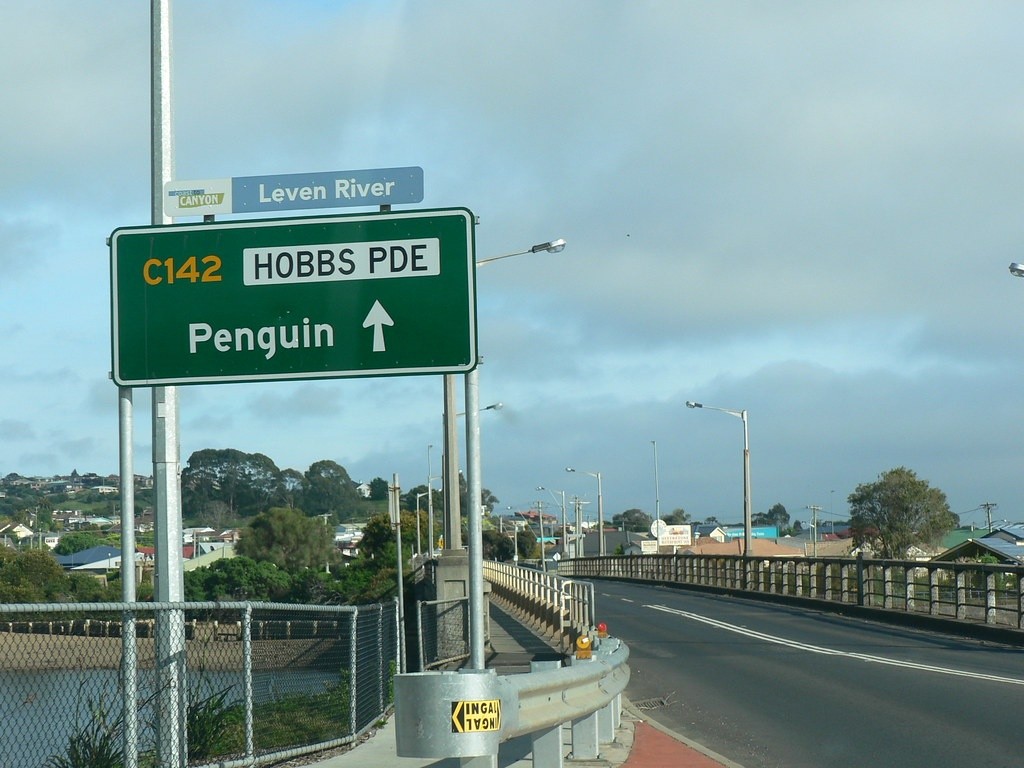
537, 485, 568, 557
416, 488, 442, 556
566, 467, 605, 556
462, 236, 568, 677
427, 441, 433, 557
441, 402, 503, 550
684, 400, 753, 556
